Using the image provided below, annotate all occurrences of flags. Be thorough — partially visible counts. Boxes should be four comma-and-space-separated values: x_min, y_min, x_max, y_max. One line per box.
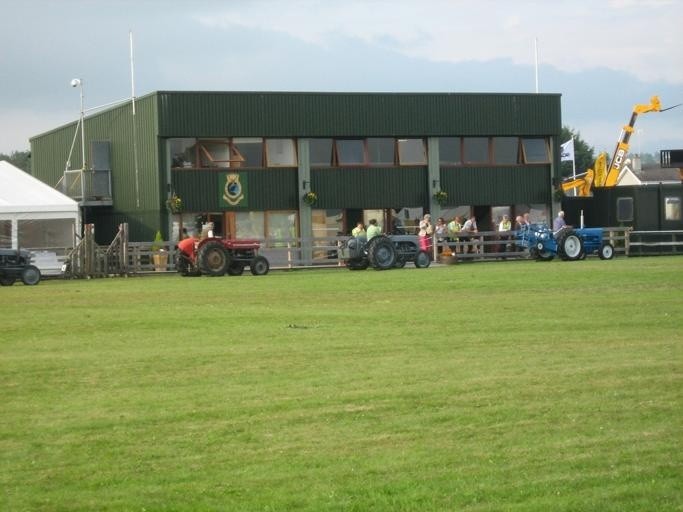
559, 138, 574, 162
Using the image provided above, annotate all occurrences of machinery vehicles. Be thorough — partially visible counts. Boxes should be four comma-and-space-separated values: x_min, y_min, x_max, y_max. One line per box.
517, 224, 614, 260
562, 96, 661, 196
341, 230, 431, 269
176, 237, 269, 275
0, 248, 40, 285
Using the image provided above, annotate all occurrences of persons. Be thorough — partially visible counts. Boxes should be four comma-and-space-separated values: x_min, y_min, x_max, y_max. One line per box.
553, 211, 566, 232
496, 215, 511, 260
523, 213, 531, 225
447, 216, 462, 252
435, 217, 448, 253
174, 228, 189, 250
462, 216, 478, 253
417, 214, 434, 253
352, 223, 366, 238
515, 216, 523, 251
366, 219, 385, 241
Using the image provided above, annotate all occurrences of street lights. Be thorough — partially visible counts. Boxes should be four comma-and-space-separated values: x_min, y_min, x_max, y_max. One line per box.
70, 77, 86, 169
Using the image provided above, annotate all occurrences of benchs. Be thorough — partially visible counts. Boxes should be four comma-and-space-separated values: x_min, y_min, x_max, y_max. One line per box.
30, 252, 65, 280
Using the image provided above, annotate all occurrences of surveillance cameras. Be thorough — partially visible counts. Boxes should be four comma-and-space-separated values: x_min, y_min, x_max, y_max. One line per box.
71, 80, 77, 87
